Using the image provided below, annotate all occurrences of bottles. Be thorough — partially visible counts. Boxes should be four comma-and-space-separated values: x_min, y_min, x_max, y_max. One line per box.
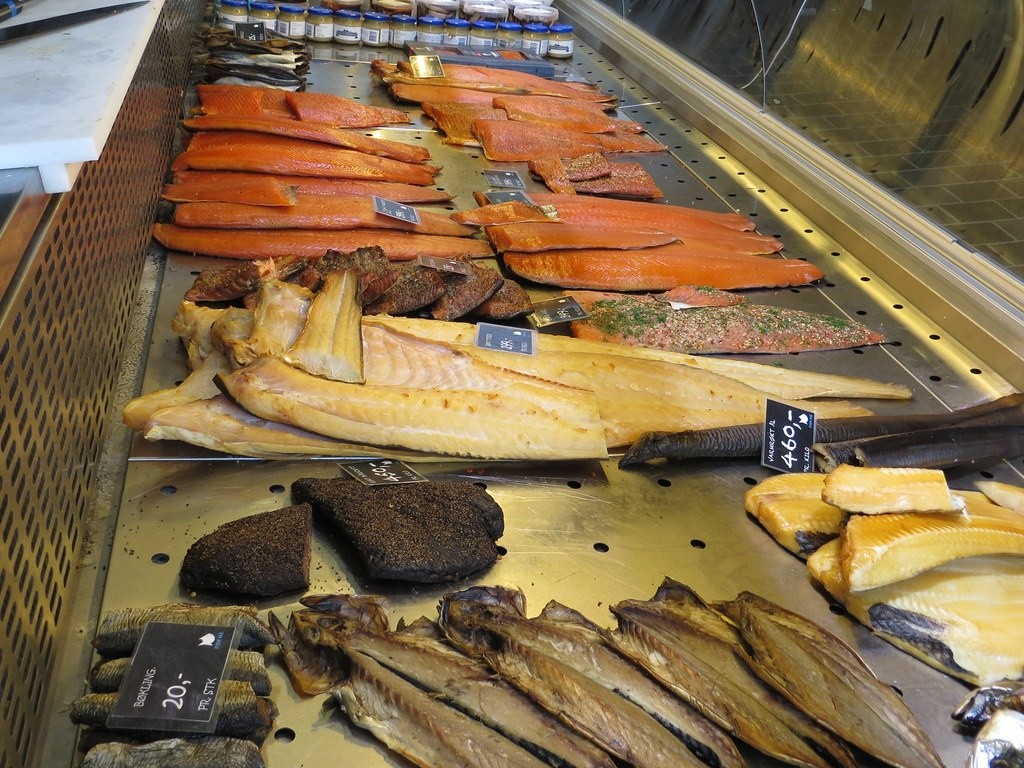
218, 0, 576, 58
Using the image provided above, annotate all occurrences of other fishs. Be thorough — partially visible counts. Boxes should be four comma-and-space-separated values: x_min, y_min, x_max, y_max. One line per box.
270, 575, 1024, 768
188, 22, 311, 91
621, 390, 1023, 472
73, 600, 280, 768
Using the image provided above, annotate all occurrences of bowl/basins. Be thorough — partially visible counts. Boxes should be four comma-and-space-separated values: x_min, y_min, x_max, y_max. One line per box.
321, 0, 363, 11
463, 5, 507, 24
458, 0, 503, 20
416, 0, 459, 22
514, 5, 558, 29
370, 0, 414, 16
505, 0, 544, 23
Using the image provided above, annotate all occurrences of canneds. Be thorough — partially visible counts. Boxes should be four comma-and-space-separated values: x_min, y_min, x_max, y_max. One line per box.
217, 0, 575, 58
